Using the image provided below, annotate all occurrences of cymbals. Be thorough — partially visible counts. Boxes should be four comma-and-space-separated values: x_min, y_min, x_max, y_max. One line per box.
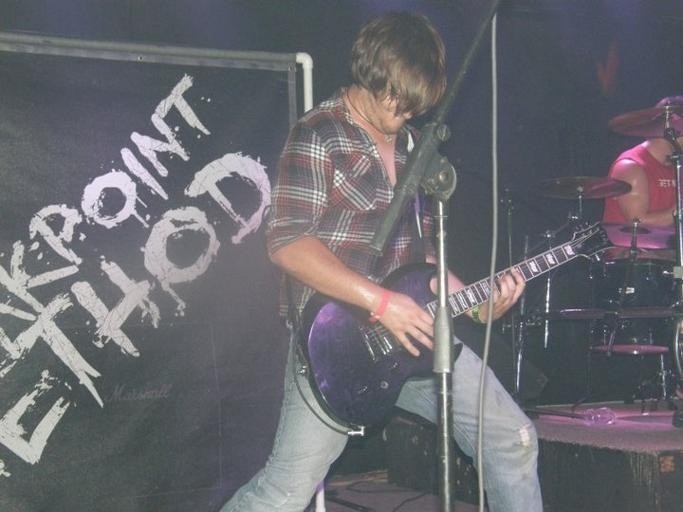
604, 226, 675, 250
528, 176, 632, 200
607, 106, 682, 137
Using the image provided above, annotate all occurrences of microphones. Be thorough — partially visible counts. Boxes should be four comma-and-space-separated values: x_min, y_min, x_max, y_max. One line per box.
577, 186, 584, 216
631, 218, 639, 249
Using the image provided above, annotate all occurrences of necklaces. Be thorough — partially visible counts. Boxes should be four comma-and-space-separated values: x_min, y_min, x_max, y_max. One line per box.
346, 87, 394, 144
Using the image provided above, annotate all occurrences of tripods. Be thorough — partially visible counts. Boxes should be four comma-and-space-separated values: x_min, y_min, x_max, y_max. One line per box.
503, 234, 598, 421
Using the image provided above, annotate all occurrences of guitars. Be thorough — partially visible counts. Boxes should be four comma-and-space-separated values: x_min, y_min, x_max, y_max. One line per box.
297, 219, 617, 434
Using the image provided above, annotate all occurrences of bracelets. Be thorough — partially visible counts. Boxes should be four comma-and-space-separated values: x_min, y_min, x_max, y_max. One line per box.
369, 288, 390, 325
472, 305, 487, 329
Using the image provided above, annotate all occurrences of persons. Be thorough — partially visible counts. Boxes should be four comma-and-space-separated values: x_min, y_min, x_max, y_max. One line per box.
604, 96, 683, 230
218, 13, 544, 512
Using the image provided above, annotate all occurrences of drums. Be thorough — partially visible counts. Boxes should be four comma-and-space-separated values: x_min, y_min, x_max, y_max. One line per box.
590, 316, 676, 353
590, 258, 682, 316
521, 250, 605, 320
